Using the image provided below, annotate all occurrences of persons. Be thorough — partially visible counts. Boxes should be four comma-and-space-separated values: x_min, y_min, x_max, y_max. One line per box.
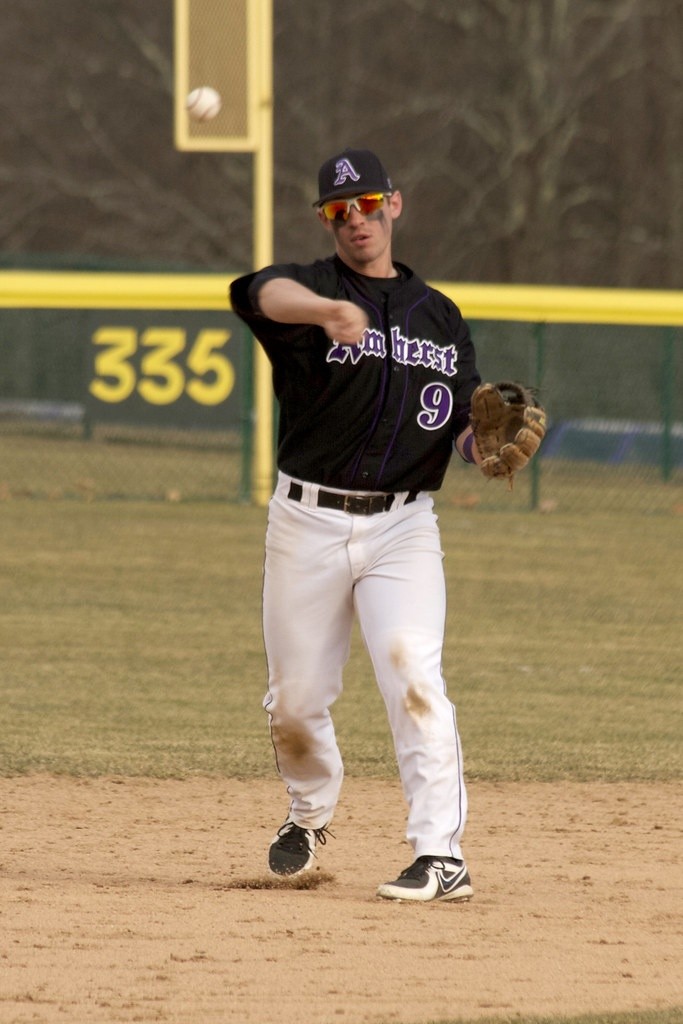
229, 149, 545, 904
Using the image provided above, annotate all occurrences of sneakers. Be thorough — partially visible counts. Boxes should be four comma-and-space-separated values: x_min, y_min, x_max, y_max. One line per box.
266, 800, 335, 881
376, 855, 474, 904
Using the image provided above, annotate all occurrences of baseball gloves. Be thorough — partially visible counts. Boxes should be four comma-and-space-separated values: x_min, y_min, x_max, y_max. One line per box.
469, 380, 548, 481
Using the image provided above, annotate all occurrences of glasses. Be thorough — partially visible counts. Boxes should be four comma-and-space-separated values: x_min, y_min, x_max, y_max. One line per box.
320, 192, 392, 221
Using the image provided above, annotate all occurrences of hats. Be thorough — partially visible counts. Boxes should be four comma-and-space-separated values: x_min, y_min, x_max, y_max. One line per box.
313, 148, 393, 206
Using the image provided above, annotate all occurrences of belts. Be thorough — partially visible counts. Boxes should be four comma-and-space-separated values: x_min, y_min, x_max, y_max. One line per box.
288, 481, 420, 514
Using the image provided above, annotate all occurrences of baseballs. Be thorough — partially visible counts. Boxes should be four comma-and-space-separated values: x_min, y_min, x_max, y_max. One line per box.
184, 86, 221, 123
